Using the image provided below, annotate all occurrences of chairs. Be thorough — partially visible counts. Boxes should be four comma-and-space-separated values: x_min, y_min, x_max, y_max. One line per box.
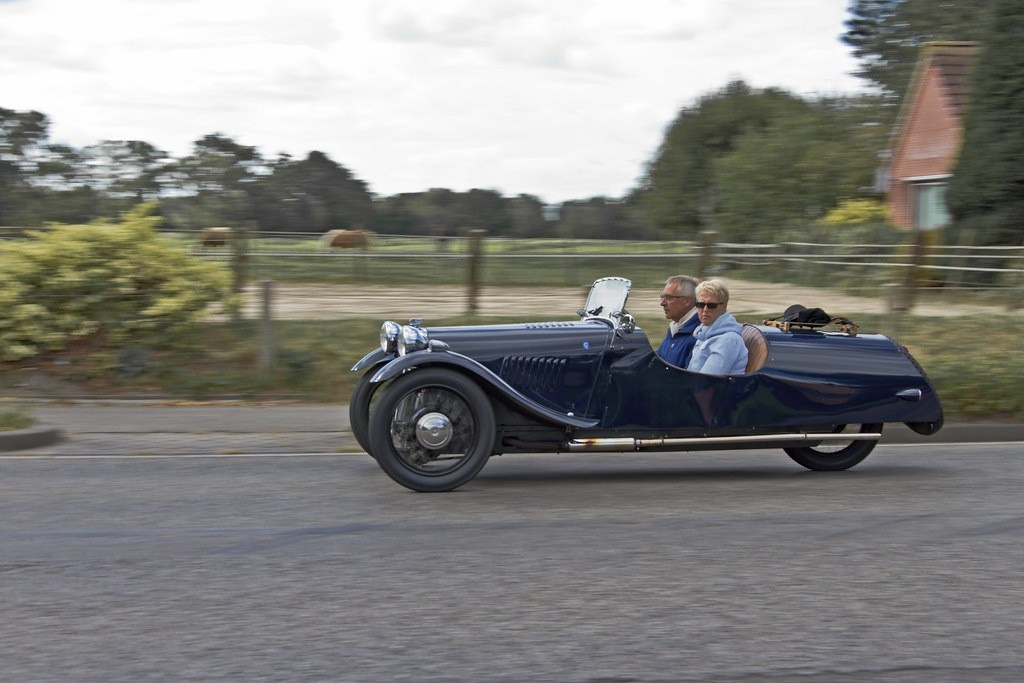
738, 324, 769, 375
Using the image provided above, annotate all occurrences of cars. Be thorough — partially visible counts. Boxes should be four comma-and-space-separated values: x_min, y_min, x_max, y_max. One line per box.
347, 274, 945, 494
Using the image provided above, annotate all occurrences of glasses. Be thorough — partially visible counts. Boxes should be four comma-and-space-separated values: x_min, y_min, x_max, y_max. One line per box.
695, 301, 722, 310
659, 295, 688, 301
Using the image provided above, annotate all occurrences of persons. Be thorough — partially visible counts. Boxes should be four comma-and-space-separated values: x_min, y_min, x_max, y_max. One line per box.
687, 280, 749, 375
655, 275, 701, 369
